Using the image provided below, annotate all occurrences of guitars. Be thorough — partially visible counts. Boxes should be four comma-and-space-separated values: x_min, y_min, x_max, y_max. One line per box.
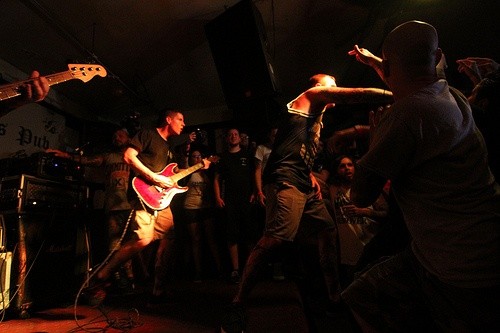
132, 154, 221, 210
0, 60, 106, 101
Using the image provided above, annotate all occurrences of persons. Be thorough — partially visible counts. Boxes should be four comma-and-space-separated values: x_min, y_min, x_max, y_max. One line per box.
97, 109, 211, 306
0, 70, 51, 118
328, 20, 500, 333
45, 128, 130, 258
233, 74, 393, 304
182, 122, 284, 285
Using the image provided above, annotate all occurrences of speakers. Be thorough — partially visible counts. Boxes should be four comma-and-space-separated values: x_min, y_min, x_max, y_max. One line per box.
1, 210, 88, 308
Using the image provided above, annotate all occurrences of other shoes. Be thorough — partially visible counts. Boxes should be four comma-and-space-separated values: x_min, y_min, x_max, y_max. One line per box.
220, 304, 248, 333
